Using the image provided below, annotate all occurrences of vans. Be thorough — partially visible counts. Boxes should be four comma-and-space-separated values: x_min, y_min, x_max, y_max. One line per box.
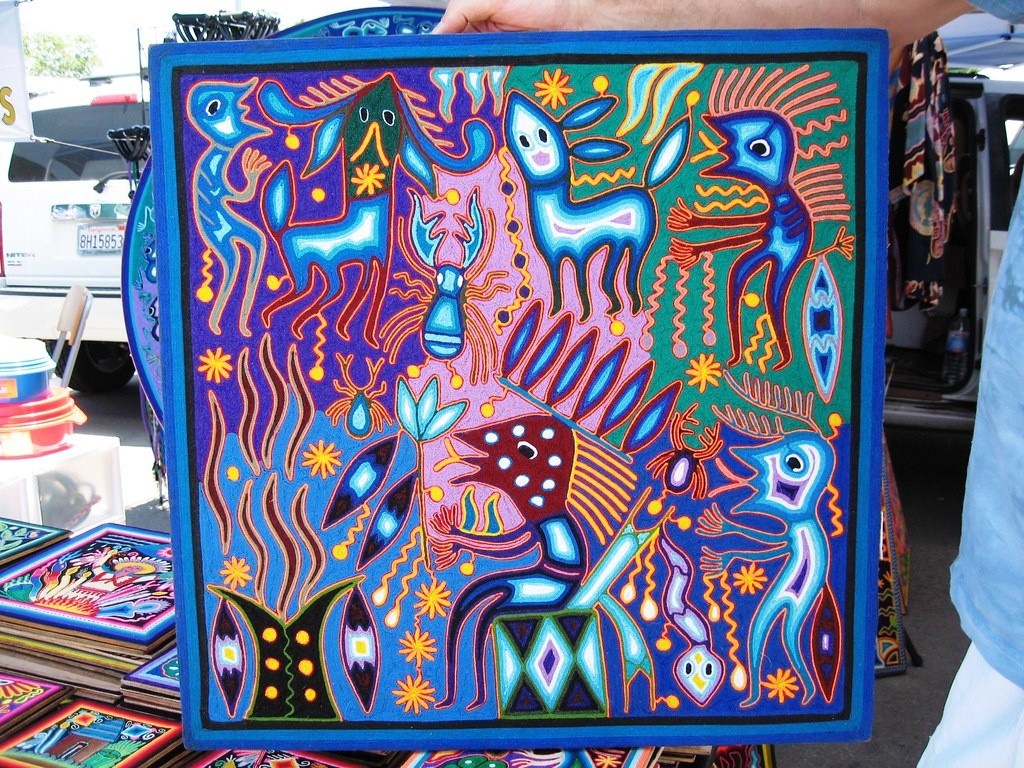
885, 56, 1023, 433
0, 86, 149, 394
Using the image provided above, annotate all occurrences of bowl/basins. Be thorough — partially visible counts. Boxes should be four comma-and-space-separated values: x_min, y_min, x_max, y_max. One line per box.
0, 337, 87, 459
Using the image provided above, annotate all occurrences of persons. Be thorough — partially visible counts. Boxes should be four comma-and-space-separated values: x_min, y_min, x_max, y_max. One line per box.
427, 1, 1024, 768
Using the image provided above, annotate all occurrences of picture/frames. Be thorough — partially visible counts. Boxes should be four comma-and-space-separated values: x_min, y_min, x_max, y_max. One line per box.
146, 28, 890, 751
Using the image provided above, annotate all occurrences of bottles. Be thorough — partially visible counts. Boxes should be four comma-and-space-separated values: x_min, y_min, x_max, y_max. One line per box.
941, 308, 969, 387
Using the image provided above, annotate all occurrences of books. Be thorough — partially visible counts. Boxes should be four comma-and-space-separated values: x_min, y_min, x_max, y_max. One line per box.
0, 487, 710, 768
